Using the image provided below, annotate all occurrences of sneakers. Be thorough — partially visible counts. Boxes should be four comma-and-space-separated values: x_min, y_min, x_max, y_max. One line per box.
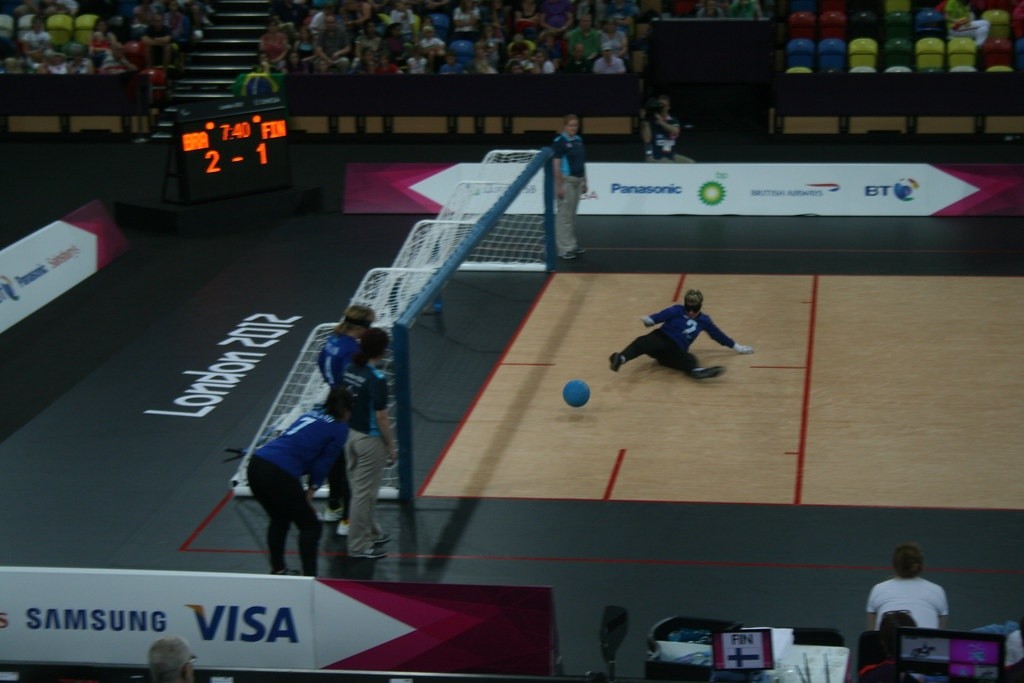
372, 534, 391, 543
347, 547, 388, 558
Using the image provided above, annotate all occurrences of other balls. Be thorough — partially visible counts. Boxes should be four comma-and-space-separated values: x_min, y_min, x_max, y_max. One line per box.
562, 380, 590, 408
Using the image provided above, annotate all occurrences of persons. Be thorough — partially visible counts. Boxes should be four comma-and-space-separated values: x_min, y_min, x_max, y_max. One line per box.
640, 96, 695, 164
341, 328, 397, 558
256, 0, 641, 74
610, 289, 752, 379
149, 636, 198, 683
865, 544, 949, 631
944, 0, 990, 48
247, 389, 355, 575
0, 0, 217, 74
317, 306, 373, 536
689, 0, 763, 18
552, 114, 588, 259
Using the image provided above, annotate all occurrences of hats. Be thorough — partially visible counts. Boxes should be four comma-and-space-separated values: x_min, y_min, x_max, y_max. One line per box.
424, 26, 433, 33
601, 42, 612, 50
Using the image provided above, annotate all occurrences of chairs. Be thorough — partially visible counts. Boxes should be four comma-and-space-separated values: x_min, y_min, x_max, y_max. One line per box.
0, 0, 1024, 80
790, 627, 889, 669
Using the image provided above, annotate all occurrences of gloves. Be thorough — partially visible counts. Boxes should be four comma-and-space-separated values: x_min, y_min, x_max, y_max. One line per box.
641, 316, 655, 326
733, 343, 753, 354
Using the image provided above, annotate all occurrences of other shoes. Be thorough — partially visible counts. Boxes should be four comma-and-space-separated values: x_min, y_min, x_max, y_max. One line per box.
324, 507, 343, 520
571, 248, 584, 253
610, 352, 621, 371
691, 366, 726, 379
271, 569, 299, 575
561, 253, 576, 258
337, 520, 350, 535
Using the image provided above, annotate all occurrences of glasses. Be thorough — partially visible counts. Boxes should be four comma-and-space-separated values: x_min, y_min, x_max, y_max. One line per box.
684, 303, 701, 313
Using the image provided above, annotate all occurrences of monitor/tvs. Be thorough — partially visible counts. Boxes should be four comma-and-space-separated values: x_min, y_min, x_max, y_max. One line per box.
896, 625, 1006, 683
